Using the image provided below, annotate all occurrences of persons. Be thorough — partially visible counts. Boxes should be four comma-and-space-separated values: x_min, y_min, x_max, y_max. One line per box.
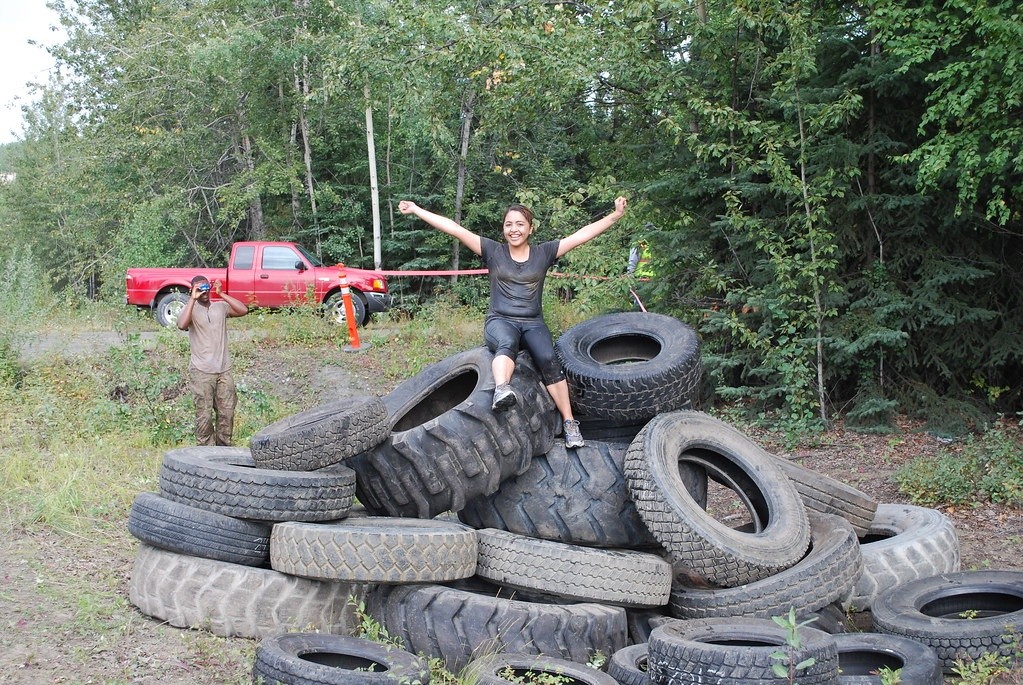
177, 275, 248, 446
398, 195, 628, 449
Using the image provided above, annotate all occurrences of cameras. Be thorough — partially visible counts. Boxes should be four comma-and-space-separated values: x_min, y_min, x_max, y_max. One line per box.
199, 283, 211, 291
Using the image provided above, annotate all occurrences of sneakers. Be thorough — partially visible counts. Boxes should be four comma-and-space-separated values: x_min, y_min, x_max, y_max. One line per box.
563, 418, 585, 448
492, 382, 518, 414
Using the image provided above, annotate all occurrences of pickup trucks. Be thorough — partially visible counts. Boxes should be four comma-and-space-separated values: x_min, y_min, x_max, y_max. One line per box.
124, 240, 391, 329
